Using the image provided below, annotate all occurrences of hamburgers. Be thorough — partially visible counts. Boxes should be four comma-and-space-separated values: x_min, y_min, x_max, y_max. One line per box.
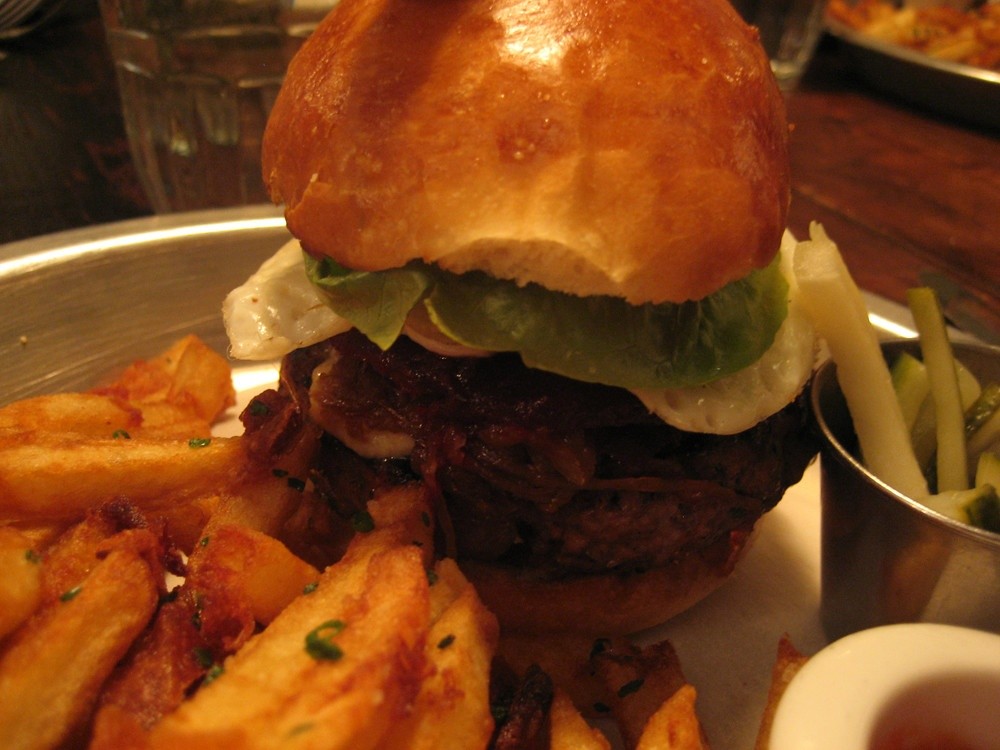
222, 0, 848, 640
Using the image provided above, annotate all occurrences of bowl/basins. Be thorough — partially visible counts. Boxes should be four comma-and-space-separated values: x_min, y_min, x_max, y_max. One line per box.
801, 16, 1000, 141
0, 202, 999, 750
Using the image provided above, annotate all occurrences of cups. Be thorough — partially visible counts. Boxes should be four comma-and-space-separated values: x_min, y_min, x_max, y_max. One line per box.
99, 0, 342, 215
812, 341, 1000, 643
729, 0, 826, 93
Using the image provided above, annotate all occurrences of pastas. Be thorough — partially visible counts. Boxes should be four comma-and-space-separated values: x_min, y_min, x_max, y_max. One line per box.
829, 0, 1000, 80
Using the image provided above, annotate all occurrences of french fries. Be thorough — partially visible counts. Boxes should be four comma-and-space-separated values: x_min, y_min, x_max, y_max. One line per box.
0, 331, 821, 750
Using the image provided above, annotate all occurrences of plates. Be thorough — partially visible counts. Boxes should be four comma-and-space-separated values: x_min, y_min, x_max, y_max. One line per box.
768, 622, 1000, 750
0, 0, 61, 39
